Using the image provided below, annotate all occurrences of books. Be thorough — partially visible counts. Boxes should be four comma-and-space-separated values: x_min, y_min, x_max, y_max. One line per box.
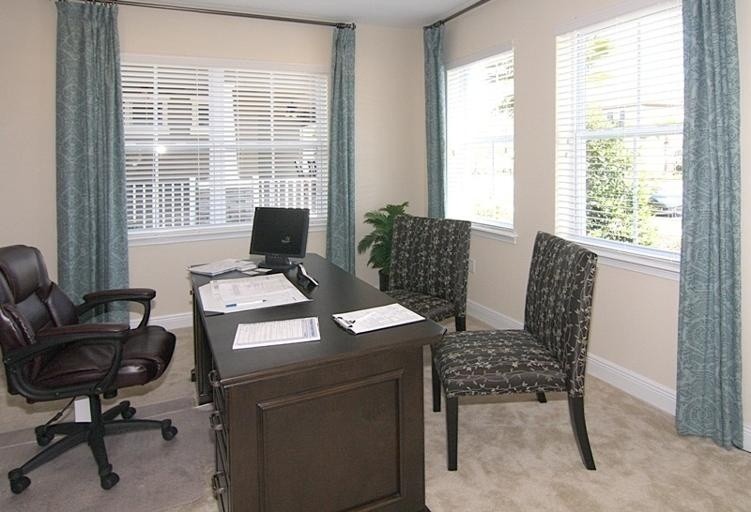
232, 315, 322, 352
188, 257, 242, 277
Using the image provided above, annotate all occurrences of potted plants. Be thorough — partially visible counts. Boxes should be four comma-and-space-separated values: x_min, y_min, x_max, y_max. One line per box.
357, 202, 410, 292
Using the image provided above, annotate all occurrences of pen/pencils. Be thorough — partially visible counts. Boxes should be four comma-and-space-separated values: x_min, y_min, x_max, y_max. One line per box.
226, 300, 268, 308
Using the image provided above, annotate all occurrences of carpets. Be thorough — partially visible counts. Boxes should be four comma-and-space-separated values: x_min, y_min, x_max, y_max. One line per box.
1, 399, 430, 512
0, 398, 216, 512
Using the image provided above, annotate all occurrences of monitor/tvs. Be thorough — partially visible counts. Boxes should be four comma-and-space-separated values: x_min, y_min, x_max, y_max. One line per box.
249, 207, 310, 273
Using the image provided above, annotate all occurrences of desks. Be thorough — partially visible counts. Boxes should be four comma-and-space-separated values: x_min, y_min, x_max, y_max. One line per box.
188, 253, 443, 512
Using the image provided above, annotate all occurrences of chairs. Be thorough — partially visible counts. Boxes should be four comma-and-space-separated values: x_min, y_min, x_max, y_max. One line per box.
0, 242, 178, 494
433, 232, 600, 470
384, 217, 470, 334
